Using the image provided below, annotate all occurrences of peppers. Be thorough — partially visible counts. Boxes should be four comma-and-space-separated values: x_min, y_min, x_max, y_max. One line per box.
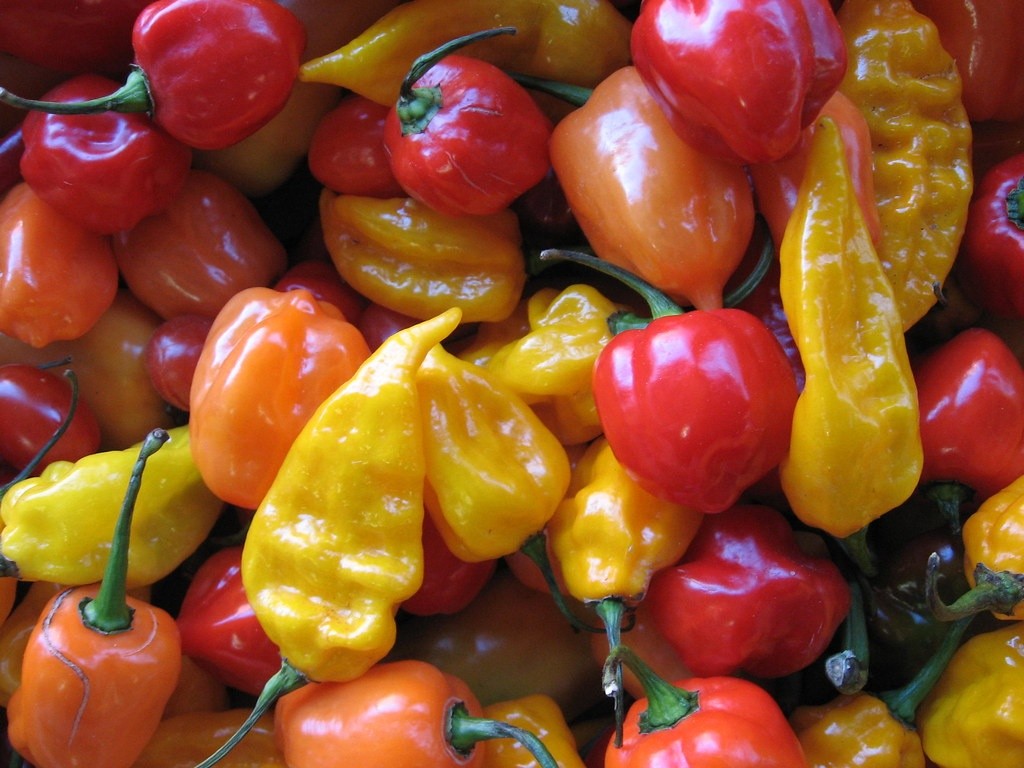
0, 0, 1024, 768
